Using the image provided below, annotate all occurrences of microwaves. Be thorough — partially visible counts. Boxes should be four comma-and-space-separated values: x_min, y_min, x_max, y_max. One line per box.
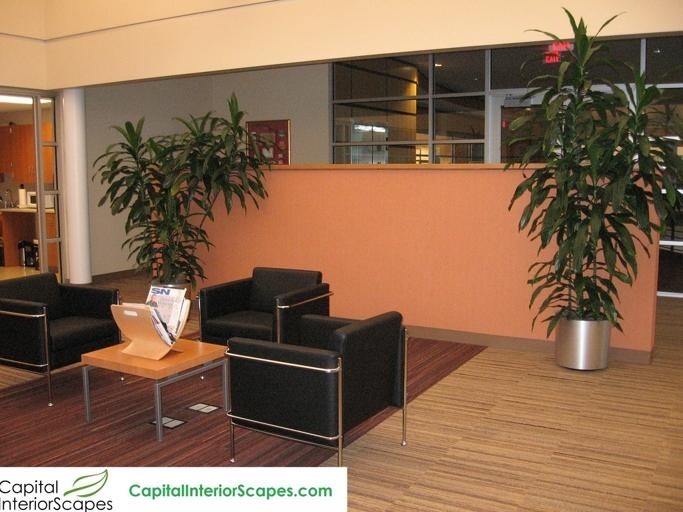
26, 191, 54, 209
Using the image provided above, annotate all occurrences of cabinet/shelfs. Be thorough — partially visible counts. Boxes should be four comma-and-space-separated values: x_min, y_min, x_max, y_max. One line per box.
0, 119, 54, 190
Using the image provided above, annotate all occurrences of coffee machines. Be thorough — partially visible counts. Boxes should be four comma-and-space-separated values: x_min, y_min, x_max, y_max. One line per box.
17, 240, 37, 266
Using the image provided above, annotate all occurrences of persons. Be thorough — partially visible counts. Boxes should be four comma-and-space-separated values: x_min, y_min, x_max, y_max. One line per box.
260, 124, 275, 159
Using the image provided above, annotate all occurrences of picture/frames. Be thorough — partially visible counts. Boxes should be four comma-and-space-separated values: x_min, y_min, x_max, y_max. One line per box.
242, 118, 292, 165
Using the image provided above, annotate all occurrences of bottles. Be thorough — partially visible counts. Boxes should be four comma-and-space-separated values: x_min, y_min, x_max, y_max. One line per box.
2, 192, 9, 208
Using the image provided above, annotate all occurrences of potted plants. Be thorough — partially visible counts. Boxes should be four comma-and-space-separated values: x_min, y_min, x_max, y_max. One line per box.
92, 92, 281, 312
501, 0, 683, 371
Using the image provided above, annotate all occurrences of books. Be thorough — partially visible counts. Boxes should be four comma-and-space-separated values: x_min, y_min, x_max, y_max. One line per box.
121, 285, 187, 347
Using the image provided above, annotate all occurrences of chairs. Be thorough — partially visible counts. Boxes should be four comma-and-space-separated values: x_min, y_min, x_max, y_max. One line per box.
0, 271, 126, 408
200, 267, 334, 356
222, 309, 407, 467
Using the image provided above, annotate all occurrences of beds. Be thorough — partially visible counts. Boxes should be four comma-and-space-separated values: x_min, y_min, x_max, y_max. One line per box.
80, 335, 230, 445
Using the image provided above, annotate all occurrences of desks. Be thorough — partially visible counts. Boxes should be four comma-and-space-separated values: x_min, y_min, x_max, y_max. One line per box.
1, 205, 58, 272
